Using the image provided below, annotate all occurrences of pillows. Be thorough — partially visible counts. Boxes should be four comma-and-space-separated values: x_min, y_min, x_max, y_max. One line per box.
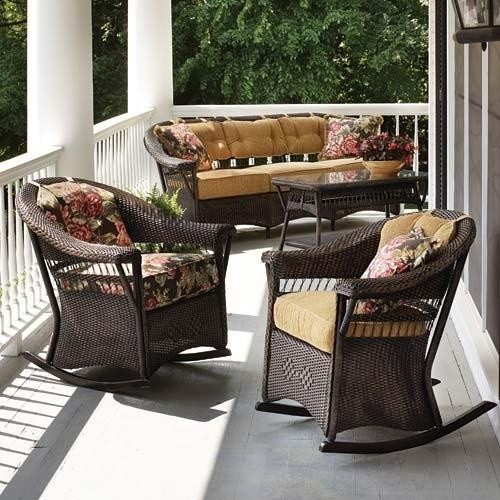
346, 228, 435, 313
151, 118, 217, 174
318, 113, 385, 161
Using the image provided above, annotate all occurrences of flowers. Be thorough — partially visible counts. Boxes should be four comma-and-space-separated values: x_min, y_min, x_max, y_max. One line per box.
356, 133, 416, 161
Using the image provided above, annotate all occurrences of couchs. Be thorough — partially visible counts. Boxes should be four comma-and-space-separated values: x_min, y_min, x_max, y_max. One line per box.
143, 113, 402, 238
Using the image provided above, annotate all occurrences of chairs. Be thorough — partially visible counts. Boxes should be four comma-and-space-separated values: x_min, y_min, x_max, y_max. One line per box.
256, 210, 500, 457
14, 176, 236, 396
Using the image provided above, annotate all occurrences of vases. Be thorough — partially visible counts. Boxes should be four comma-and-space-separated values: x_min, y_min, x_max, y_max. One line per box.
355, 158, 413, 178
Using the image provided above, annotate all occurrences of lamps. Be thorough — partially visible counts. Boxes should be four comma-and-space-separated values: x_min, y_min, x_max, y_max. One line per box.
450, 0, 499, 50
